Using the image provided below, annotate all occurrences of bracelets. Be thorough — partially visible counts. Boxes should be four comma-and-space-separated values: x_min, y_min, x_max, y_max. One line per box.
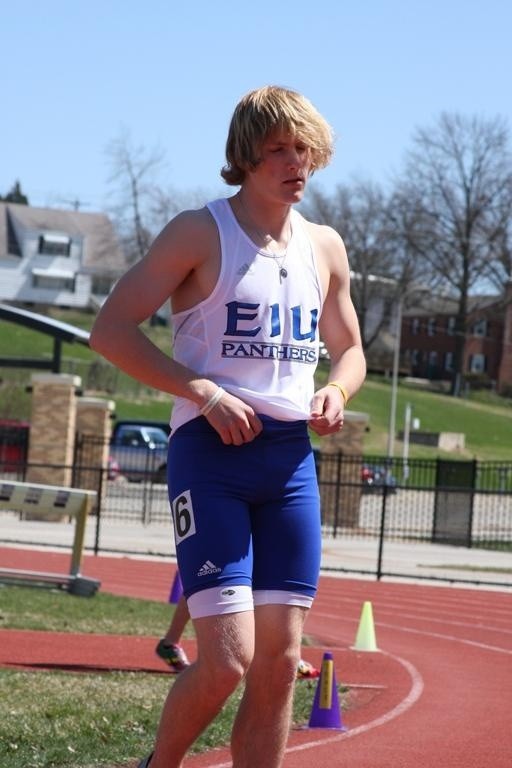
199, 385, 224, 415
327, 381, 347, 405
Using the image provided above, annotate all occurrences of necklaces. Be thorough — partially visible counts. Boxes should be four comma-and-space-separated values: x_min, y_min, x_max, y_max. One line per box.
236, 192, 288, 279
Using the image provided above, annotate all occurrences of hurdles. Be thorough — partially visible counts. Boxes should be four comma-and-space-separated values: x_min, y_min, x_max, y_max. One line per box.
1, 480, 100, 599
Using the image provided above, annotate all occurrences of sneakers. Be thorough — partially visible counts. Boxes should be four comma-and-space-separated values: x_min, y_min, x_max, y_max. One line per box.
296, 661, 319, 679
155, 638, 192, 670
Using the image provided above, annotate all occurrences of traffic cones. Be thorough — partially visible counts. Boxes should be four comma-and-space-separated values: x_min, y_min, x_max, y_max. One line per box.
303, 650, 347, 732
348, 598, 382, 654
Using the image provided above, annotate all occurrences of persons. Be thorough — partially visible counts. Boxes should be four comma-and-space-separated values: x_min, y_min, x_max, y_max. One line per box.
85, 83, 375, 768
153, 568, 317, 680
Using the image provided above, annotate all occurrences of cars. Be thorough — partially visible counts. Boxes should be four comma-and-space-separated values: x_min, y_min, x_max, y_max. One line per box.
360, 464, 398, 495
109, 422, 170, 484
0, 419, 121, 482
